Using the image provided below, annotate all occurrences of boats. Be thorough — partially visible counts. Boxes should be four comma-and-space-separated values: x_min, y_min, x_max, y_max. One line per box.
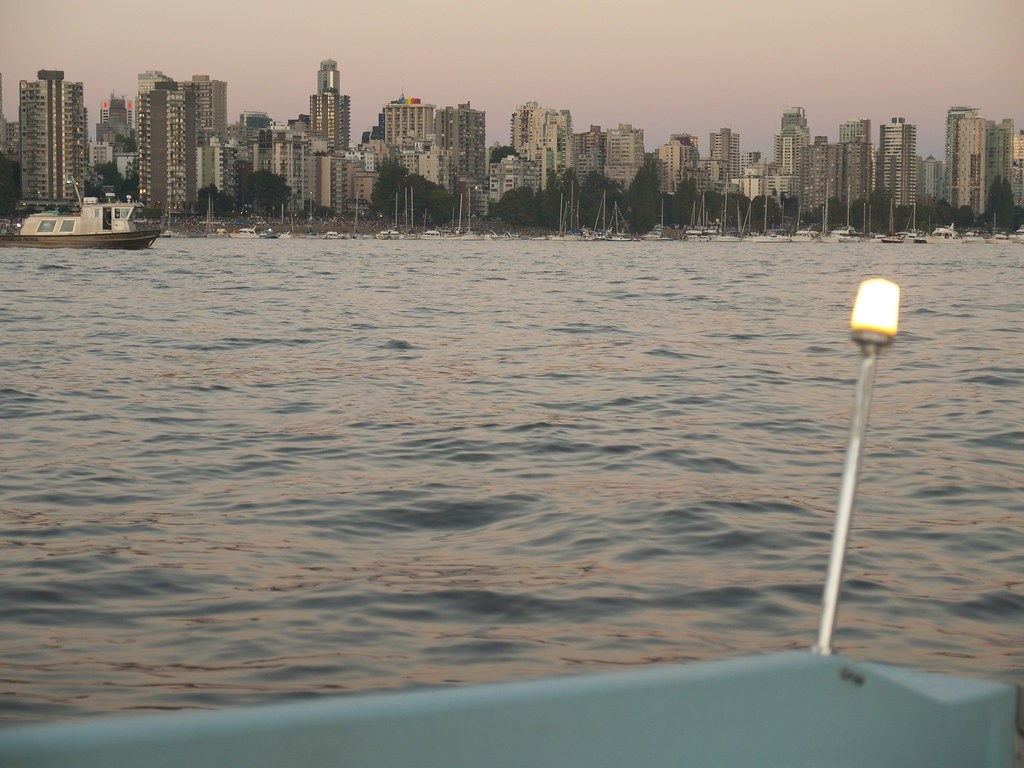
490, 235, 547, 241
213, 228, 292, 241
1, 193, 162, 252
297, 231, 375, 241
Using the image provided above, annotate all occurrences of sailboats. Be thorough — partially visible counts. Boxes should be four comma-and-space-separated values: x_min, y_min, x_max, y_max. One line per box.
379, 186, 485, 241
551, 172, 1024, 245
